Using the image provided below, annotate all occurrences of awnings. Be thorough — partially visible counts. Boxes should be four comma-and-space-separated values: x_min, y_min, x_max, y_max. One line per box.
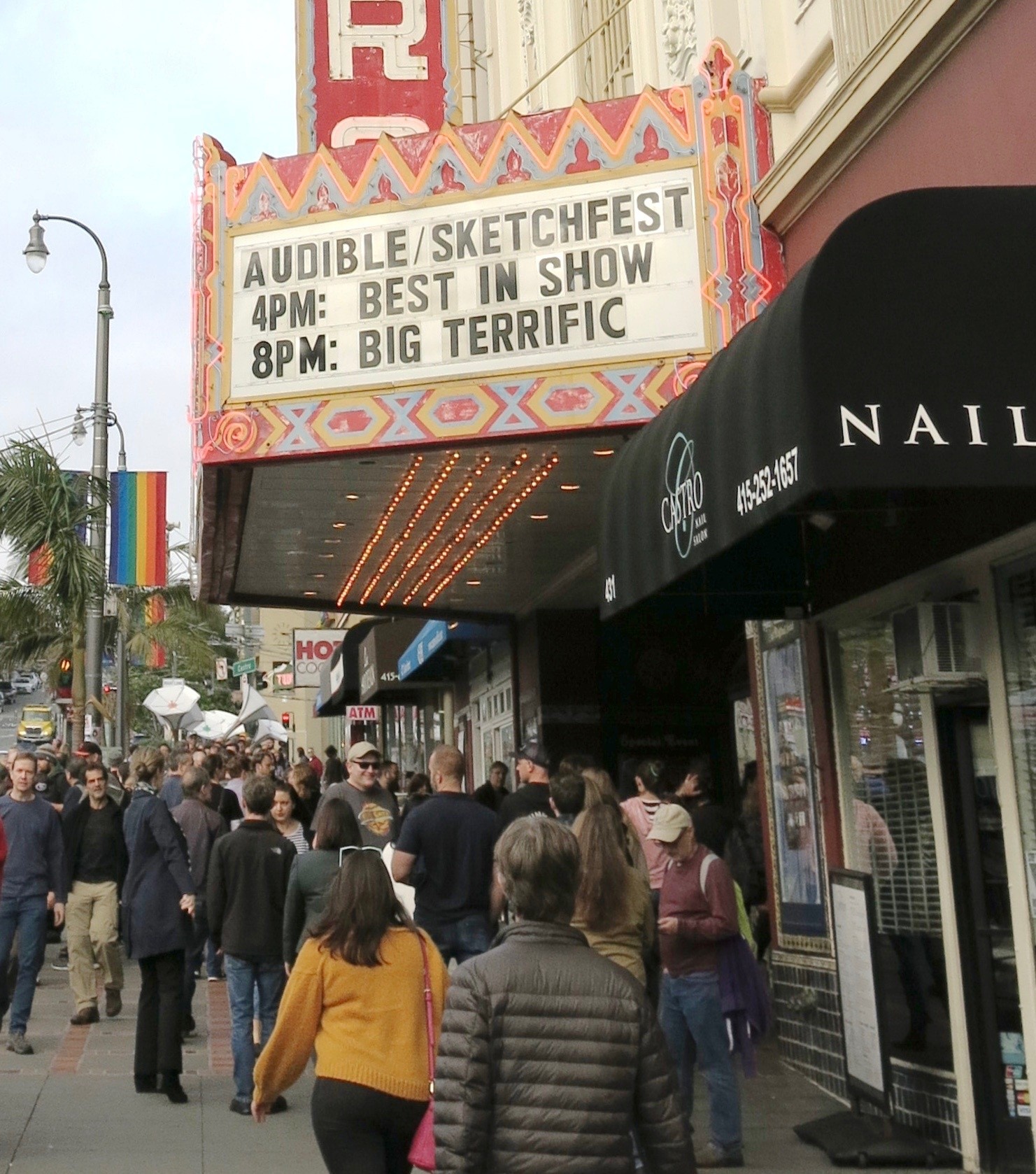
357, 620, 450, 706
602, 185, 1036, 622
313, 616, 443, 718
398, 620, 504, 682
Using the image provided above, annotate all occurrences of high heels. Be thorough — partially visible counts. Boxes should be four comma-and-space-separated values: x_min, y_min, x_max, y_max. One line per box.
156, 1072, 187, 1104
135, 1075, 160, 1093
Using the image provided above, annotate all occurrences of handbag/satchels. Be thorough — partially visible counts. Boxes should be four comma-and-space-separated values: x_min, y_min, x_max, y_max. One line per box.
409, 1080, 438, 1171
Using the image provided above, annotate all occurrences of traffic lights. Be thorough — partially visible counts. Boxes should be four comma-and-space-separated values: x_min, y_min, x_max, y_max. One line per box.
282, 714, 290, 729
60, 660, 71, 672
105, 685, 110, 693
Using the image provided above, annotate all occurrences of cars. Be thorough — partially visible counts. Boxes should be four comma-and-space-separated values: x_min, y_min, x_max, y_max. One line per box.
0, 672, 41, 713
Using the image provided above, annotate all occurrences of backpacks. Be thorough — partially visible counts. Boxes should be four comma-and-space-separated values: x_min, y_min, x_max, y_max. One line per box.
666, 854, 760, 959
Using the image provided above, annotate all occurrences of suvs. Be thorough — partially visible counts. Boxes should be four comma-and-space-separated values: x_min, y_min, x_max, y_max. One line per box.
17, 704, 56, 744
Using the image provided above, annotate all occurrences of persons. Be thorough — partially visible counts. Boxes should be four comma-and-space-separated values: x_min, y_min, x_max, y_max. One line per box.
250, 848, 450, 1174
311, 741, 400, 857
783, 754, 943, 932
207, 776, 297, 1115
569, 803, 656, 1168
121, 746, 196, 1104
169, 766, 227, 981
48, 765, 130, 1025
0, 752, 70, 1055
283, 798, 363, 1065
251, 783, 312, 1058
646, 804, 745, 1168
391, 744, 507, 967
433, 817, 698, 1174
470, 744, 770, 965
0, 732, 431, 970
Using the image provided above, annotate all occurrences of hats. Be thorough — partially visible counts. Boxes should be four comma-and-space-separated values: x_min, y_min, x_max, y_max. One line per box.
74, 742, 101, 757
645, 803, 693, 843
508, 741, 554, 767
347, 741, 384, 760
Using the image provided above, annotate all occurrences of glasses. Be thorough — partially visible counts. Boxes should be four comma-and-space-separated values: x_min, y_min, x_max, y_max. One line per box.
653, 829, 688, 848
350, 760, 380, 770
339, 845, 382, 869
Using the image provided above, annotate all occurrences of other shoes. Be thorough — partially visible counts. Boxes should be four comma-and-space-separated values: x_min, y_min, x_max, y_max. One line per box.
71, 1007, 100, 1024
230, 1098, 251, 1115
51, 960, 69, 969
6, 1032, 32, 1055
265, 1096, 287, 1114
694, 1146, 745, 1168
105, 987, 122, 1017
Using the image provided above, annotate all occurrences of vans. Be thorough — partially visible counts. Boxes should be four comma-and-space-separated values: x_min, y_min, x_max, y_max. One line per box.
82, 714, 102, 738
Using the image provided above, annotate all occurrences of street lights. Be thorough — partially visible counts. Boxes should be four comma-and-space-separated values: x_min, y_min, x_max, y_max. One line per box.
21, 209, 134, 760
